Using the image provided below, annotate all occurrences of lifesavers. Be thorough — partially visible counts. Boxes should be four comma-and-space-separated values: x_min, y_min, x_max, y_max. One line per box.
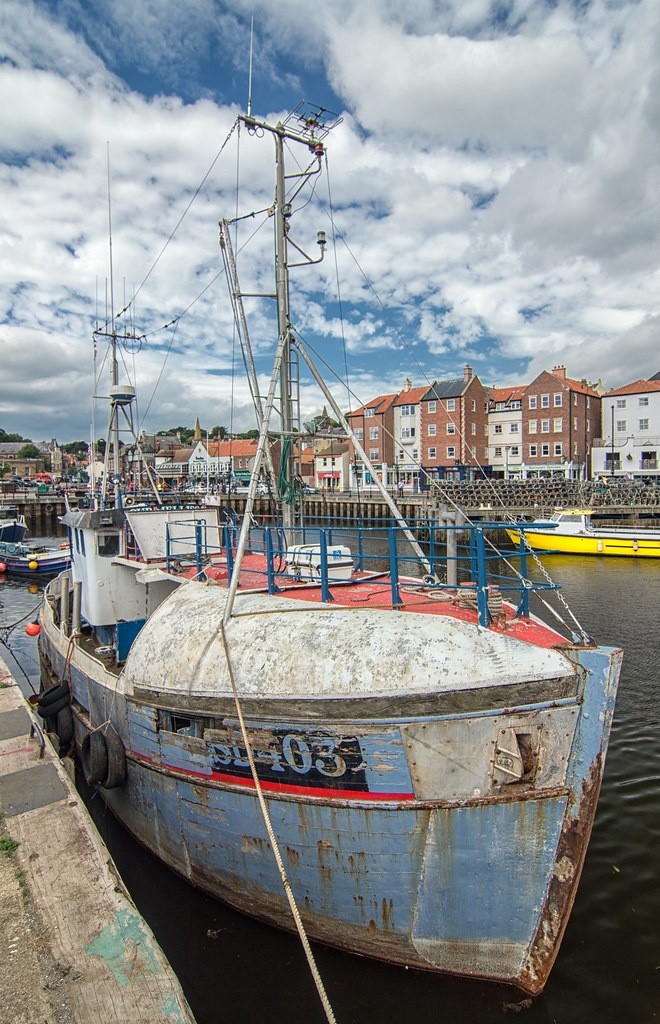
81, 730, 108, 784
126, 497, 133, 504
45, 504, 54, 512
59, 543, 71, 550
37, 680, 73, 745
100, 733, 125, 790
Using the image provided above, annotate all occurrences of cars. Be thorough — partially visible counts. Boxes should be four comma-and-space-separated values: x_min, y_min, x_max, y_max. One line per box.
186, 486, 207, 494
0, 479, 46, 487
256, 480, 275, 496
303, 485, 319, 495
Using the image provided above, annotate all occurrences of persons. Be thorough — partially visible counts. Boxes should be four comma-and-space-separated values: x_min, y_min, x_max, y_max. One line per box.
161, 482, 168, 491
398, 479, 405, 498
209, 483, 214, 495
230, 482, 234, 493
178, 482, 183, 491
219, 482, 223, 494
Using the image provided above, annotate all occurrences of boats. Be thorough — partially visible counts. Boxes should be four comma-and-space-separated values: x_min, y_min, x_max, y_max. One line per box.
500, 506, 660, 559
0, 539, 71, 574
0, 504, 29, 544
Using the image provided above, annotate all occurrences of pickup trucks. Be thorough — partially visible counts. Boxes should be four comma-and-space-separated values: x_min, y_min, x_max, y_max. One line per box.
95, 481, 114, 493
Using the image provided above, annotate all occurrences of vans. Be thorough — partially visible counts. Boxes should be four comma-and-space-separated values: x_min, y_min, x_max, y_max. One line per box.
34, 474, 51, 483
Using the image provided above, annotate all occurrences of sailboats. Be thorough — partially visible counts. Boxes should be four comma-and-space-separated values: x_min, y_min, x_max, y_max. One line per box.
27, 13, 627, 1006
